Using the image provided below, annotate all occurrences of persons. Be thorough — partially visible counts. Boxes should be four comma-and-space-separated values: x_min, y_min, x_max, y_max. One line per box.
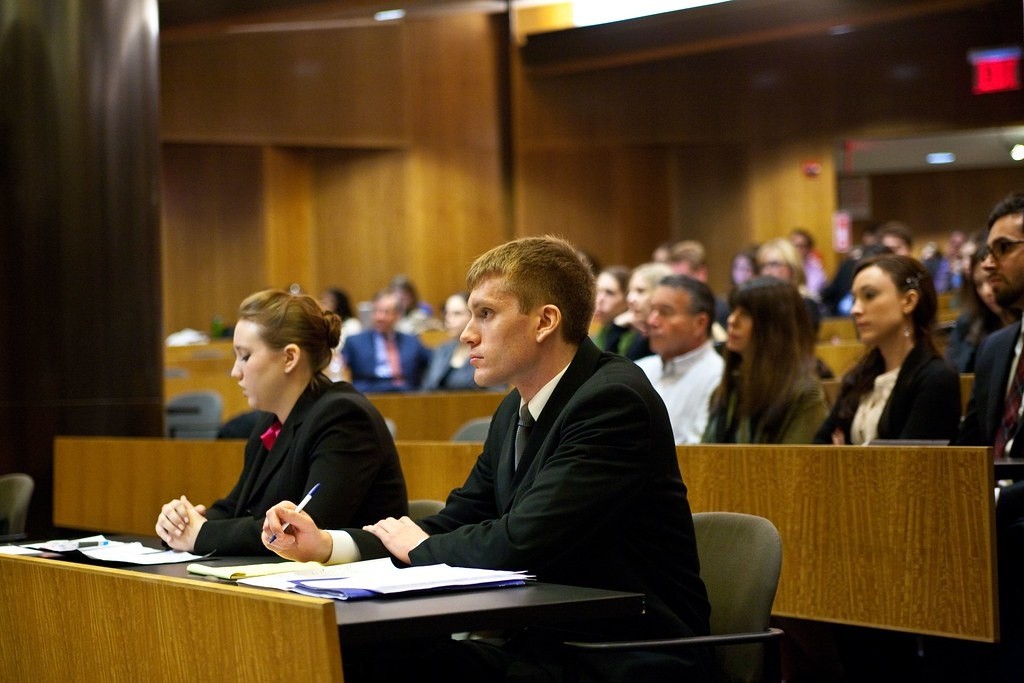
309, 190, 1024, 534
260, 236, 712, 683
155, 289, 408, 556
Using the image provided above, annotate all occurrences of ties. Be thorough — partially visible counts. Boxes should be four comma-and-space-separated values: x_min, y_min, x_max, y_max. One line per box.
513, 403, 535, 473
994, 340, 1024, 462
385, 336, 407, 388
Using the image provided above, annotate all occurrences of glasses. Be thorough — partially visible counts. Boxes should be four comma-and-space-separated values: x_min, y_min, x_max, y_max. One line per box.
976, 237, 1024, 262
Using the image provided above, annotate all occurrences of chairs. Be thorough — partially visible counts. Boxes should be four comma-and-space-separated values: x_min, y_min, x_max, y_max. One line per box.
166, 393, 220, 439
0, 474, 35, 543
556, 512, 781, 683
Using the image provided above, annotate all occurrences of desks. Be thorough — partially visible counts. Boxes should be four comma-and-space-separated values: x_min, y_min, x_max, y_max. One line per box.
0, 282, 1019, 683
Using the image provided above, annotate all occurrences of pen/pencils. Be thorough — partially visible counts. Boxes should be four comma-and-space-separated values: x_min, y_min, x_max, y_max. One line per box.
269, 482, 322, 544
